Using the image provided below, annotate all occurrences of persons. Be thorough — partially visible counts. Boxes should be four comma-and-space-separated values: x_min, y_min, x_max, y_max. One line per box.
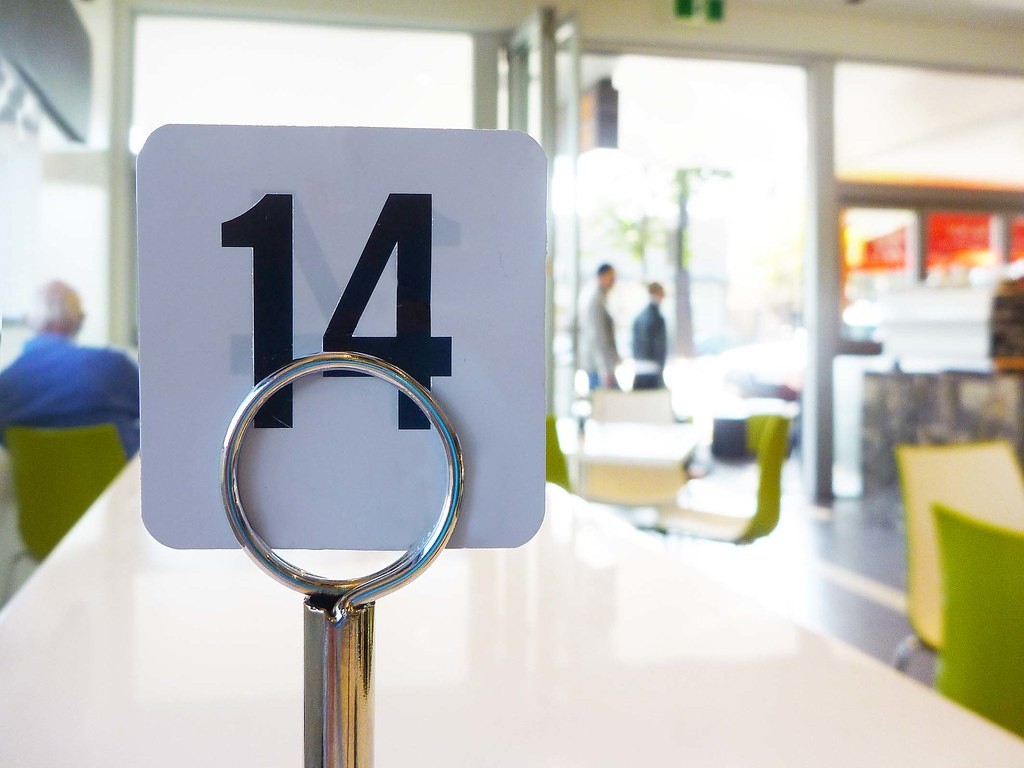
632, 284, 691, 423
1, 281, 141, 460
580, 265, 623, 433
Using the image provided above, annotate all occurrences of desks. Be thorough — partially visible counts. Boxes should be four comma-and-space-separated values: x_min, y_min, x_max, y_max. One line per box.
556, 416, 697, 469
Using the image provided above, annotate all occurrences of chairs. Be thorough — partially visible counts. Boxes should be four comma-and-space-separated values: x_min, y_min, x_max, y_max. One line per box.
544, 416, 571, 491
4, 423, 125, 602
932, 505, 1024, 739
580, 388, 692, 540
892, 437, 1024, 673
656, 408, 791, 545
